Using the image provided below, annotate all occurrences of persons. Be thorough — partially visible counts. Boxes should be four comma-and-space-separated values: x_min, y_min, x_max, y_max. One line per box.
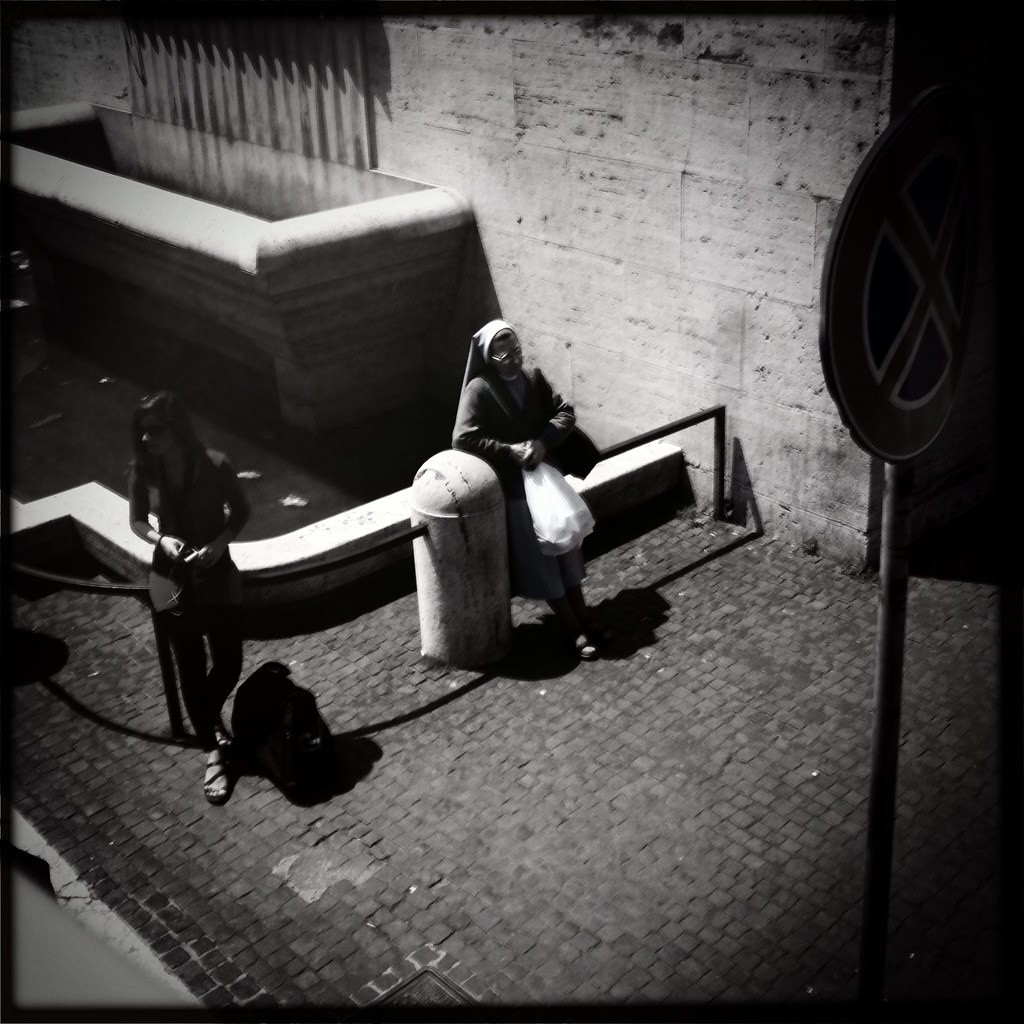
129, 389, 251, 805
452, 318, 598, 659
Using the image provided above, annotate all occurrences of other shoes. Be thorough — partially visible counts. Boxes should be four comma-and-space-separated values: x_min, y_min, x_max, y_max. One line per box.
564, 625, 602, 661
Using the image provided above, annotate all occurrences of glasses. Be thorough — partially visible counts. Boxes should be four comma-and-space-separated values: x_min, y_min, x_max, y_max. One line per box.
139, 423, 170, 439
490, 343, 523, 366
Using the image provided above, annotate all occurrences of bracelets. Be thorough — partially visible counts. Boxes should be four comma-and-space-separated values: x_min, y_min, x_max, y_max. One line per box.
158, 534, 165, 544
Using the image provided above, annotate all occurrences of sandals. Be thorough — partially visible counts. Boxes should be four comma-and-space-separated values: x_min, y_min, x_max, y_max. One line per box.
211, 719, 234, 750
200, 752, 227, 803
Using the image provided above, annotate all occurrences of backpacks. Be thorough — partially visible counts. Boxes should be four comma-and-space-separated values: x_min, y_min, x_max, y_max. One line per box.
230, 661, 332, 776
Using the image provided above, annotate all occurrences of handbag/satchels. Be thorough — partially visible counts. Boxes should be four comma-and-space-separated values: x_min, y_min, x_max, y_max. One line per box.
520, 461, 595, 557
526, 365, 601, 481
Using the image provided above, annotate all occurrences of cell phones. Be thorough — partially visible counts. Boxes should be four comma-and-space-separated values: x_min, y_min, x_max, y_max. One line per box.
182, 548, 198, 563
